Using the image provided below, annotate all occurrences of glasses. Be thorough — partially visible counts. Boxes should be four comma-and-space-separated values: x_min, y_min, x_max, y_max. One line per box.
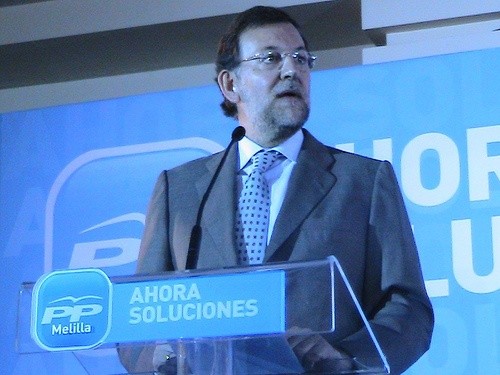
227, 48, 318, 72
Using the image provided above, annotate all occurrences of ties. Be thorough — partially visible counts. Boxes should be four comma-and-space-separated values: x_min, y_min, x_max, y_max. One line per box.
232, 149, 285, 264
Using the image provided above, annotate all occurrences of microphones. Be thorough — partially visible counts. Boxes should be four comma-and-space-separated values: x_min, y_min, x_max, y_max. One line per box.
185, 125, 246, 270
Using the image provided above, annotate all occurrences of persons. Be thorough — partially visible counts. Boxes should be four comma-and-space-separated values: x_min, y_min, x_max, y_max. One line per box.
117, 6, 434, 375
